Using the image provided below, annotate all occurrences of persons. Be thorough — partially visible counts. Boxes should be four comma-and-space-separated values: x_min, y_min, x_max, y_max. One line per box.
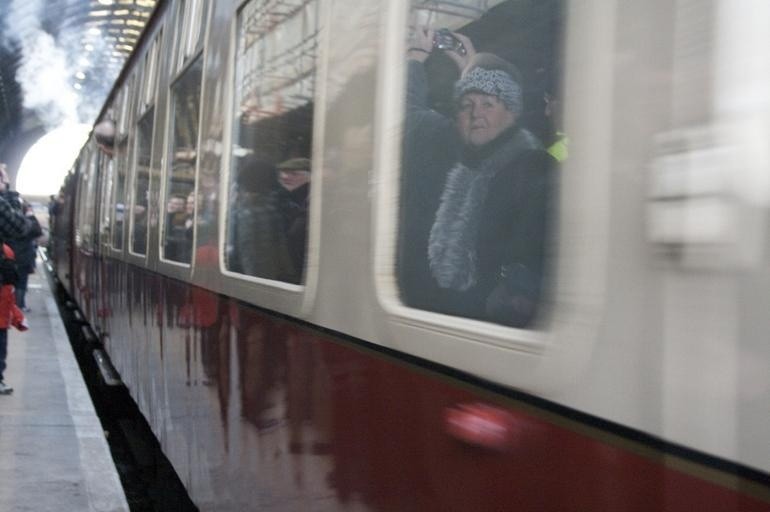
82, 187, 206, 328
272, 142, 310, 286
427, 50, 552, 327
0, 162, 45, 395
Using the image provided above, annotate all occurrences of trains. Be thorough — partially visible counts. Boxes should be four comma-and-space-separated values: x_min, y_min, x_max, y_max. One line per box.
47, 0, 770, 512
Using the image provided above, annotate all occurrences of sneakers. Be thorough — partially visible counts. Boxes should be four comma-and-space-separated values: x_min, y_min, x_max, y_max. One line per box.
0, 382, 13, 394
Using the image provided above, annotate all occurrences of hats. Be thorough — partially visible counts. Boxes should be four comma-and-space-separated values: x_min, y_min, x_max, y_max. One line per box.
453, 53, 524, 116
277, 158, 311, 170
